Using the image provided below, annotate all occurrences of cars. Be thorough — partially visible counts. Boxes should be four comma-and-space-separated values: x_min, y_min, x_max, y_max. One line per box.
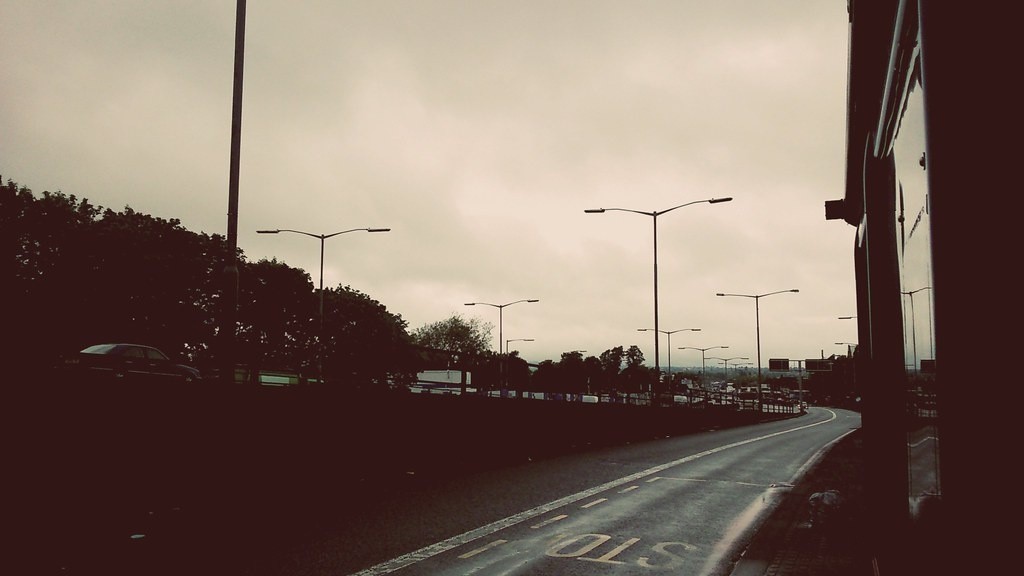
74, 345, 202, 385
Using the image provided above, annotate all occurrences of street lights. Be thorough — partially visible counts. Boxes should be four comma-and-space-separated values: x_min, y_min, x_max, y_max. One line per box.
705, 356, 754, 391
638, 328, 703, 398
506, 339, 536, 388
464, 298, 540, 390
585, 197, 733, 400
256, 227, 392, 388
678, 345, 729, 399
715, 288, 801, 424
